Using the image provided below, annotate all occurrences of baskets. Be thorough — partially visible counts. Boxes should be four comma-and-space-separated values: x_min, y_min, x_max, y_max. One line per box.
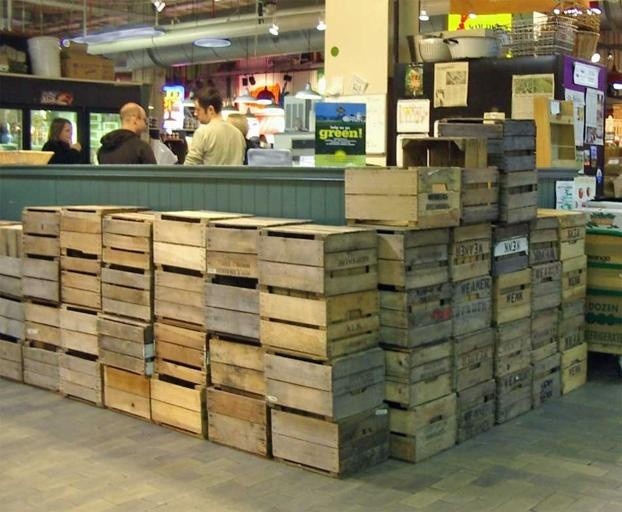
497, 2, 600, 59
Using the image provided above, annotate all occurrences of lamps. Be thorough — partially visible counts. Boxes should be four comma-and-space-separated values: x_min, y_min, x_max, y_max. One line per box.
269, 16, 279, 35
316, 11, 326, 31
151, 0, 165, 11
212, 63, 324, 86
420, 10, 429, 21
554, 0, 602, 16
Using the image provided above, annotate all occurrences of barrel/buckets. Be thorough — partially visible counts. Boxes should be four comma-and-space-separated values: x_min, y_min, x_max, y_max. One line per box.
26, 36, 63, 77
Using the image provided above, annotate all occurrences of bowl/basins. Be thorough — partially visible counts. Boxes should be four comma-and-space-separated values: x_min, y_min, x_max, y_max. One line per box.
405, 31, 451, 65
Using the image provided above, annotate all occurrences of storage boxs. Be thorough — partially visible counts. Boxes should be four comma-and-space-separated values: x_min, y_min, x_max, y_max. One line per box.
62, 57, 114, 80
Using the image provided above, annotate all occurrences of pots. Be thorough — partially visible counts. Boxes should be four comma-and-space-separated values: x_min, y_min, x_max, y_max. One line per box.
441, 28, 502, 60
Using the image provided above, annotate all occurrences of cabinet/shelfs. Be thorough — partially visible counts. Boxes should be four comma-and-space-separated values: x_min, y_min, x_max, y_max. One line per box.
514, 96, 577, 169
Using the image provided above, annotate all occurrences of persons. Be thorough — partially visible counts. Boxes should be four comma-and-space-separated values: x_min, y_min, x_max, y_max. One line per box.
1, 119, 34, 147
96, 103, 157, 164
41, 117, 83, 164
183, 87, 248, 165
